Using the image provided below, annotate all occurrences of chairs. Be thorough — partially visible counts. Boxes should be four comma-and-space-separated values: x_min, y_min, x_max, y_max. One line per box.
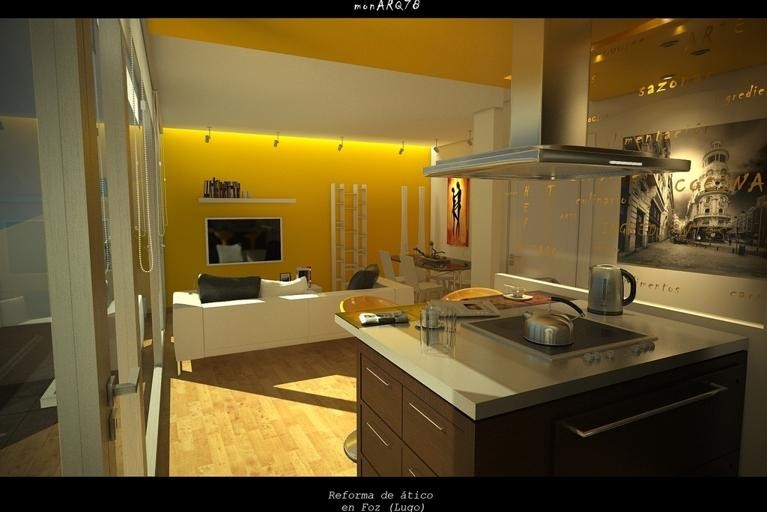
439, 287, 503, 303
338, 294, 401, 466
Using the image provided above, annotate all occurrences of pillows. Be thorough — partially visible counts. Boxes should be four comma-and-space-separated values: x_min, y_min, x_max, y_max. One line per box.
345, 269, 379, 290
258, 276, 309, 302
194, 270, 261, 305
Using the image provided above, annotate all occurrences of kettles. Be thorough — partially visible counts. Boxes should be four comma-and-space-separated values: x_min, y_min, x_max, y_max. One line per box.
584, 263, 638, 317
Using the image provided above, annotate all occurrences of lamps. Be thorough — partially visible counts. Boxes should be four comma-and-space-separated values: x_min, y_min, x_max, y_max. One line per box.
433, 138, 440, 153
204, 124, 212, 144
337, 136, 346, 152
467, 129, 474, 146
273, 130, 280, 148
398, 140, 405, 154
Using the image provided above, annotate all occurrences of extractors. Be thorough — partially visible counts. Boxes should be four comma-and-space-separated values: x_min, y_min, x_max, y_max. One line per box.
419, 18, 693, 184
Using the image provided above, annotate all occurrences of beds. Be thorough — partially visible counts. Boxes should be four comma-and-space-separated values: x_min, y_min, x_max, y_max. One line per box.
377, 248, 472, 307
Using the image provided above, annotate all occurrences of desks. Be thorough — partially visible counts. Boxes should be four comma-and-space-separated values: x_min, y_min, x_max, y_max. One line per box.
306, 283, 323, 293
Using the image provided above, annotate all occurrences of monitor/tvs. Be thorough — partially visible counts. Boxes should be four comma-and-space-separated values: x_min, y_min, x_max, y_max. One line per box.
204, 217, 283, 267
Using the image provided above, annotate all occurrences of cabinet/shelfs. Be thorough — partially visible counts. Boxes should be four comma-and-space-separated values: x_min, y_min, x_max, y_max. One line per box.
354, 333, 750, 479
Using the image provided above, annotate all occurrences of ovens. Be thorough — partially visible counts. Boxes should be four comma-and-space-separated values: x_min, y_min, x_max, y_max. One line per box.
545, 375, 729, 479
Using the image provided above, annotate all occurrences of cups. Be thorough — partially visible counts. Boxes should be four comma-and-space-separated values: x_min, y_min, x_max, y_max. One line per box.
417, 302, 458, 357
202, 176, 249, 198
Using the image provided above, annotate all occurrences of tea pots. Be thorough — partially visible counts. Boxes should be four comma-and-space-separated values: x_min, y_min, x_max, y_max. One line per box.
520, 295, 586, 347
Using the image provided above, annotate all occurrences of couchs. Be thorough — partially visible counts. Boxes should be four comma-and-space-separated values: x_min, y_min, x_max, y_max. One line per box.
0, 293, 147, 388
170, 263, 416, 376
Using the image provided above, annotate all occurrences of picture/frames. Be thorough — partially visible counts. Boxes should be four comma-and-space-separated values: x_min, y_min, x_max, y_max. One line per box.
279, 272, 291, 282
294, 266, 313, 287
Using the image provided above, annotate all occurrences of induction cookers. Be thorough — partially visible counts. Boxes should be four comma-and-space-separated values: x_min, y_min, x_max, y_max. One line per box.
457, 307, 659, 363
